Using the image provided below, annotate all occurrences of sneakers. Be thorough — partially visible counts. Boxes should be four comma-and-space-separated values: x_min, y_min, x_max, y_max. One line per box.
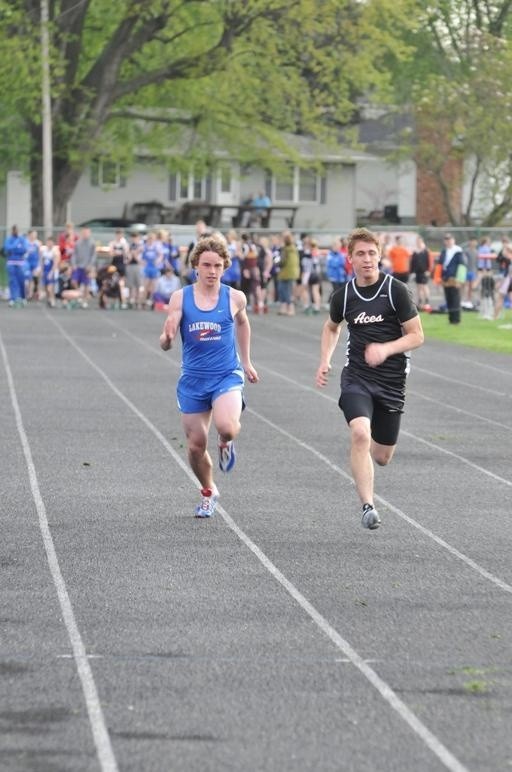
195, 484, 221, 518
218, 433, 235, 472
361, 503, 381, 530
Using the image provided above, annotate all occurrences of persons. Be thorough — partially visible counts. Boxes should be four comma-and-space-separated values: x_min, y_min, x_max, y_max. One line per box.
0, 192, 511, 330
159, 238, 259, 518
315, 227, 426, 530
